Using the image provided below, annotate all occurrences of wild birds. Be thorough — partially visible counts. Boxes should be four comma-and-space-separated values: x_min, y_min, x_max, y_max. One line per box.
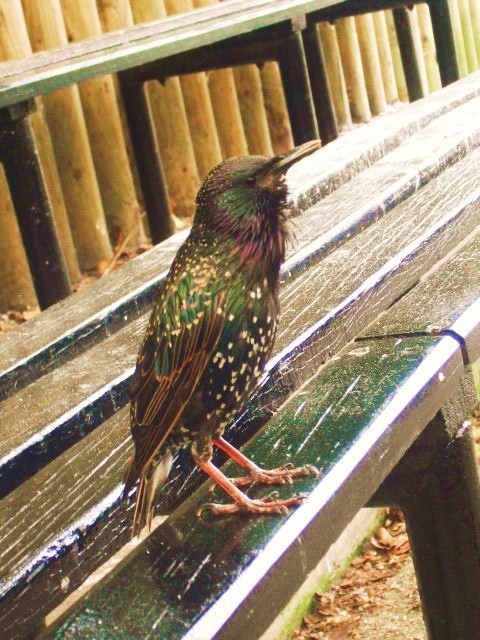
119, 139, 323, 541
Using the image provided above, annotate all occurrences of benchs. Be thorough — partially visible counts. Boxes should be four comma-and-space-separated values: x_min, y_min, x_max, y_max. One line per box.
1, 1, 471, 313
0, 72, 480, 637
